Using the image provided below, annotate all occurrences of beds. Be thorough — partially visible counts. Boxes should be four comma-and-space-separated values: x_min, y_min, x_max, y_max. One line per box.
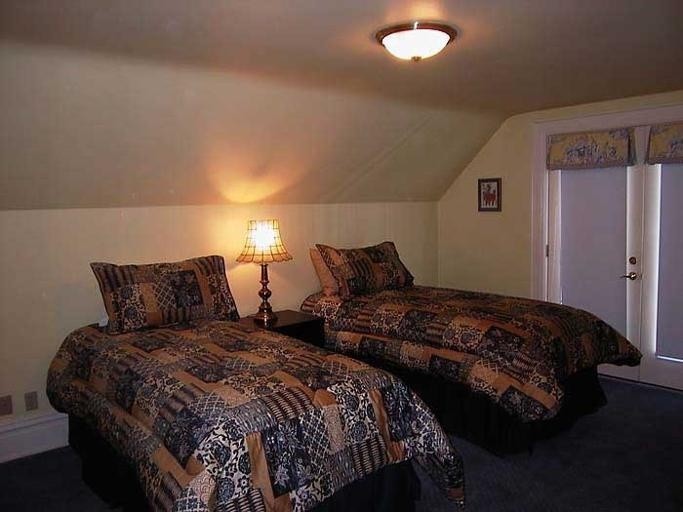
45, 317, 468, 511
300, 286, 643, 458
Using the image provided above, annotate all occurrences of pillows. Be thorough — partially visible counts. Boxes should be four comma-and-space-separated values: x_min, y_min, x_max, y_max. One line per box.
309, 248, 339, 298
315, 239, 415, 299
90, 255, 240, 336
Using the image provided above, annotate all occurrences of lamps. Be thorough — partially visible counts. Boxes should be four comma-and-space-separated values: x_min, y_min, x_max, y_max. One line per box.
376, 22, 456, 64
234, 218, 293, 326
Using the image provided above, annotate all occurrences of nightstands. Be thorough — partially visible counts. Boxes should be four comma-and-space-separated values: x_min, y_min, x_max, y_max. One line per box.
241, 310, 325, 350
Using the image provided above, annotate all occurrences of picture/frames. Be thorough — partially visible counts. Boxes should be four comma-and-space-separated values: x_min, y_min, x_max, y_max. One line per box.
477, 177, 502, 212
